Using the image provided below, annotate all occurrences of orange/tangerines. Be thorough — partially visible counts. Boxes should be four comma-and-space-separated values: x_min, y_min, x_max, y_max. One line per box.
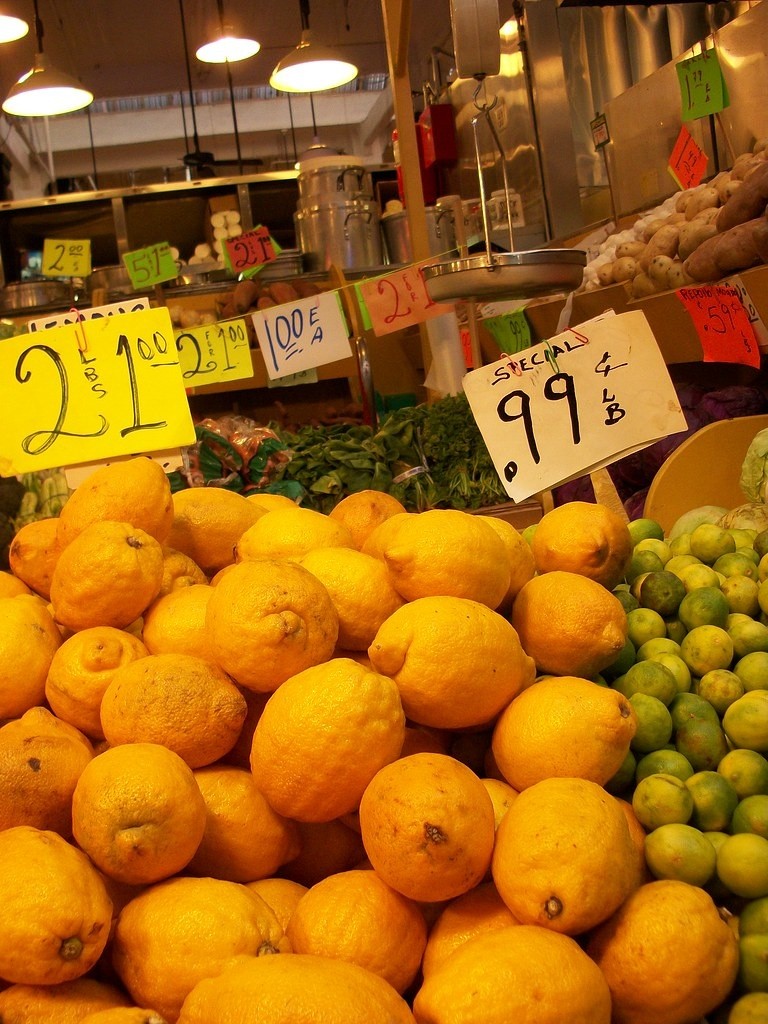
600, 519, 768, 1024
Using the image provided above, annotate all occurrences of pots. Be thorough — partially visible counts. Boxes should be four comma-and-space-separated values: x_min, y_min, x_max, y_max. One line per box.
294, 200, 381, 273
296, 191, 374, 208
298, 164, 368, 199
382, 207, 455, 264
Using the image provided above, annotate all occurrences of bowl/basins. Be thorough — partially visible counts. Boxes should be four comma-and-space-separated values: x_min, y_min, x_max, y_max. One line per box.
419, 249, 588, 303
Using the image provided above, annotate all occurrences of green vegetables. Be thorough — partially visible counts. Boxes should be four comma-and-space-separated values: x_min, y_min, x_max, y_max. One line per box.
279, 394, 507, 512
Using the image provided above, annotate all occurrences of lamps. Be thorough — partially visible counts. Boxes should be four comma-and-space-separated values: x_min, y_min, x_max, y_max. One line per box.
2, 0, 94, 117
196, 0, 261, 64
269, 0, 359, 92
296, 92, 340, 163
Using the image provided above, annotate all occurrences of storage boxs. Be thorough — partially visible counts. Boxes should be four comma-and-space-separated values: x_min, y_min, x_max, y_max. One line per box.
457, 208, 650, 368
626, 262, 768, 365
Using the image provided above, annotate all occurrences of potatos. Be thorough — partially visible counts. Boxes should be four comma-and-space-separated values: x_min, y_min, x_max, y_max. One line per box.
218, 281, 327, 350
581, 140, 768, 294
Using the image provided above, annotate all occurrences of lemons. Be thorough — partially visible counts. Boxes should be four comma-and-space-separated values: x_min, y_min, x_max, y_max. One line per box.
1, 457, 738, 1024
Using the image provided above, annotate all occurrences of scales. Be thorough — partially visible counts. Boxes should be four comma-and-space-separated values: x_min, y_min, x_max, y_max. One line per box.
417, 0, 588, 305
